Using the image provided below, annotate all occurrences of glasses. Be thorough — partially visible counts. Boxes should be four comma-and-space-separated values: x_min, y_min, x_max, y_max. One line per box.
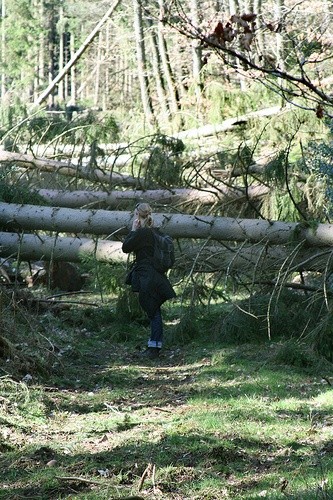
135, 203, 140, 211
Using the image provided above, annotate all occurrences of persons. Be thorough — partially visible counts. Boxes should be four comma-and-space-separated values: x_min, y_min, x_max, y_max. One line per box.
122, 202, 174, 358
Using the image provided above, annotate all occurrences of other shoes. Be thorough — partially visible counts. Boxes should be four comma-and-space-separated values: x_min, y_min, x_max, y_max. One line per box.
141, 347, 161, 359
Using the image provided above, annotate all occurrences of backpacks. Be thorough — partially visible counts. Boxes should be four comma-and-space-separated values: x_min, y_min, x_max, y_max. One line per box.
142, 228, 176, 274
